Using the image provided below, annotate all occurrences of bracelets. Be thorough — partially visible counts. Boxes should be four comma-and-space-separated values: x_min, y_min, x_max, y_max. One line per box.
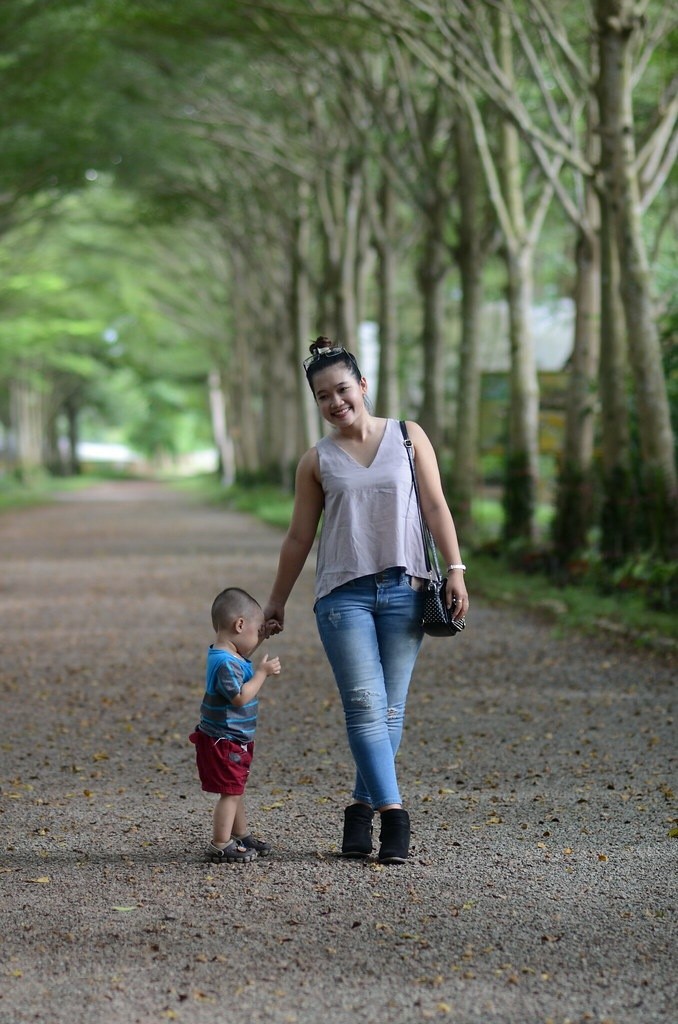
448, 565, 467, 573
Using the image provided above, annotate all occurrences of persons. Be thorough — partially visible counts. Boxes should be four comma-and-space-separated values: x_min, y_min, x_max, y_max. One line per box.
189, 588, 282, 863
262, 337, 470, 864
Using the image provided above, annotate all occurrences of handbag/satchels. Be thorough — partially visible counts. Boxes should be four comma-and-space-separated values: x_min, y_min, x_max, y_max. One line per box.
422, 577, 466, 637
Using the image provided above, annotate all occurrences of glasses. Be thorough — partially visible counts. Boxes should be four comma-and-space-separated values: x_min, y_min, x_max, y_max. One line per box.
303, 347, 362, 376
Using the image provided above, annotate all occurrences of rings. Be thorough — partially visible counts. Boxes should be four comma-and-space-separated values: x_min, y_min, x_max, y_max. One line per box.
456, 600, 462, 603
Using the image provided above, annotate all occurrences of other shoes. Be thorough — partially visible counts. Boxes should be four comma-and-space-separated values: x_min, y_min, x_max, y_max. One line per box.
379, 809, 410, 863
342, 803, 374, 859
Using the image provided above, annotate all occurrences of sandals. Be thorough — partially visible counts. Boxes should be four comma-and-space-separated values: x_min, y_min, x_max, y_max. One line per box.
204, 834, 272, 863
